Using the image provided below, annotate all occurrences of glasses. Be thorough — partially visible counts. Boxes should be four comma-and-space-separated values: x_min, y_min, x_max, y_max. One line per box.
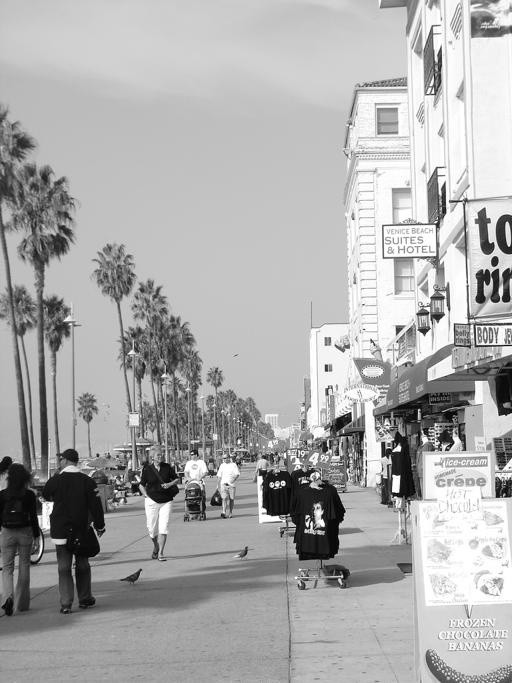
190, 454, 195, 456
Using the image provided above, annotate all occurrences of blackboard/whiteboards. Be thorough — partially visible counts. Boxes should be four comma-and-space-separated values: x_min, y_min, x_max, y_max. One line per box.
321, 461, 348, 493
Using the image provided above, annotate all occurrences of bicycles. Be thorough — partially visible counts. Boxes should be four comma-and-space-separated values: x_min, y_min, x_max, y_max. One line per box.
0, 522, 45, 571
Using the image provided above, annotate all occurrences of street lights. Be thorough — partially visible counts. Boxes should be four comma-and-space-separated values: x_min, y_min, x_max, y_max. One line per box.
61, 312, 83, 452
124, 336, 140, 472
158, 361, 273, 461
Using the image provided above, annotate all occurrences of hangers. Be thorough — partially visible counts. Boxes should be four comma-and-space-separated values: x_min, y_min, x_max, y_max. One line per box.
309, 470, 325, 490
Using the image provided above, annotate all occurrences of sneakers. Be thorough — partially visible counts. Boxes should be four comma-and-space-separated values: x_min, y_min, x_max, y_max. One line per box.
79, 597, 96, 609
4, 598, 13, 616
158, 555, 167, 561
62, 607, 71, 614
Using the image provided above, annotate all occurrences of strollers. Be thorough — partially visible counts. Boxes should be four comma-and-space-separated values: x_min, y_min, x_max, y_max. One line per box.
183, 476, 208, 522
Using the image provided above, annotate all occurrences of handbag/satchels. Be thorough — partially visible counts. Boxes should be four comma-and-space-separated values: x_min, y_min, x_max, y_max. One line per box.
164, 482, 178, 498
67, 524, 100, 558
210, 489, 222, 506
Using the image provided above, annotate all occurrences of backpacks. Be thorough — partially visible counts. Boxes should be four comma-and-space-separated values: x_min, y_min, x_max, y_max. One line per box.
0, 489, 29, 529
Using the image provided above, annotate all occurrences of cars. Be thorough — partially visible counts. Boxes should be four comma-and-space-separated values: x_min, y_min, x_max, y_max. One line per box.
80, 466, 108, 483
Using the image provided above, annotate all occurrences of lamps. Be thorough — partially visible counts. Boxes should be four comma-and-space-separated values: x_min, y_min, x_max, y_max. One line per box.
429, 282, 450, 323
416, 302, 433, 336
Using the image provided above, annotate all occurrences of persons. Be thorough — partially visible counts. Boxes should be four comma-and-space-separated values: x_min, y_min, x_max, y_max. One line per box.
216, 454, 240, 518
0, 456, 13, 491
382, 432, 463, 501
0, 463, 40, 616
231, 450, 280, 478
138, 449, 179, 561
43, 449, 106, 612
184, 450, 209, 513
207, 456, 215, 477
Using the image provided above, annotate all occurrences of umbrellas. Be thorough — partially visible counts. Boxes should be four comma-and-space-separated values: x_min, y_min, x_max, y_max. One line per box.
86, 457, 115, 469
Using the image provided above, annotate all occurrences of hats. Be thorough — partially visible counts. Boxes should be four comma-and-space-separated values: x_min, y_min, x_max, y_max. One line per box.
56, 449, 79, 463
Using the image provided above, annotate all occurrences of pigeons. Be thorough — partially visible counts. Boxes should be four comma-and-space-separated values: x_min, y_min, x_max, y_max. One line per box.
232, 546, 248, 560
120, 568, 142, 584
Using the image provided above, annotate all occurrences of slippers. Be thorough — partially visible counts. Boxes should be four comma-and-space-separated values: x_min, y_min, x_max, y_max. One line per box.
152, 551, 158, 559
